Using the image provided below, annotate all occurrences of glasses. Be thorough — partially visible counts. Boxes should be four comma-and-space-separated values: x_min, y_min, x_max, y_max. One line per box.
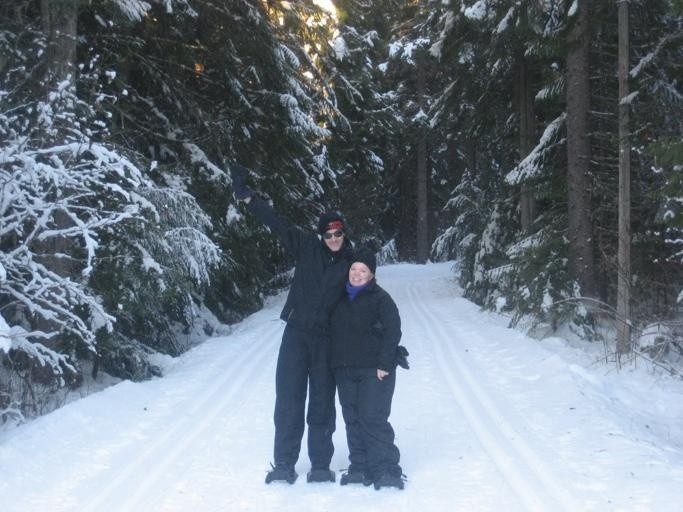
323, 231, 344, 239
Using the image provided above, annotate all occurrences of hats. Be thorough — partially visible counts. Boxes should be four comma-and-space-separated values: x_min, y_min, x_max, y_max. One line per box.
319, 212, 344, 233
349, 248, 376, 277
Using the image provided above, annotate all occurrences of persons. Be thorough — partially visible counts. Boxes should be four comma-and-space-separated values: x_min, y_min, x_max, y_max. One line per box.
326, 244, 410, 493
225, 158, 354, 484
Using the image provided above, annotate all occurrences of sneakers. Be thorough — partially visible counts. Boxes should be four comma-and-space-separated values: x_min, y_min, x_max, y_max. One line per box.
310, 468, 332, 480
272, 461, 296, 481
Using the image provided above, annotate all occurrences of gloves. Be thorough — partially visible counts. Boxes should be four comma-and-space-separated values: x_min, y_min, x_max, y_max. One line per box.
227, 167, 251, 200
